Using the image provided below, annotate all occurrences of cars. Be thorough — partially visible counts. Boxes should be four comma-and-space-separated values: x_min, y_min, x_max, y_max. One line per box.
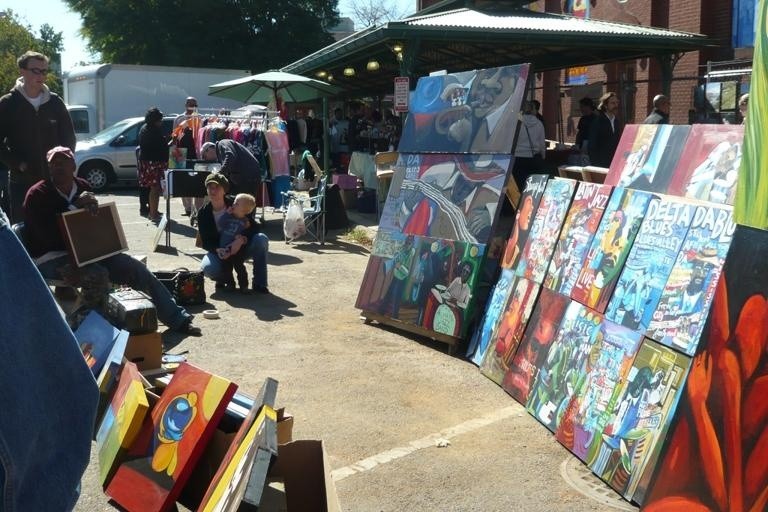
70, 117, 176, 188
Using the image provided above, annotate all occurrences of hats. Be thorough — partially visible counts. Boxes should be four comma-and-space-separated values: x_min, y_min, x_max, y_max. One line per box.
45, 145, 75, 163
204, 173, 229, 192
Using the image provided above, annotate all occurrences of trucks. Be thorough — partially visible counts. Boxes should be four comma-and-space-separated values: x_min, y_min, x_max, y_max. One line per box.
61, 67, 263, 141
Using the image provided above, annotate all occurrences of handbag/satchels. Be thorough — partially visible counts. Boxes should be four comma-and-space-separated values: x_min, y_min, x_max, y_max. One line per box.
152, 267, 206, 306
534, 152, 545, 173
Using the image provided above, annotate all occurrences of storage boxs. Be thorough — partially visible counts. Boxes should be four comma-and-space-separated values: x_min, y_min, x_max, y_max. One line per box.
144, 385, 294, 483
124, 331, 162, 371
257, 439, 342, 512
339, 189, 354, 209
333, 175, 357, 190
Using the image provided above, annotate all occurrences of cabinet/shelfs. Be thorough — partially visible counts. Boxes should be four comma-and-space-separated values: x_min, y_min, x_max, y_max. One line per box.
280, 191, 327, 244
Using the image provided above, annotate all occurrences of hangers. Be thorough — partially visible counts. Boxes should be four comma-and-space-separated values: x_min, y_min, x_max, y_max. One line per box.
193, 106, 280, 131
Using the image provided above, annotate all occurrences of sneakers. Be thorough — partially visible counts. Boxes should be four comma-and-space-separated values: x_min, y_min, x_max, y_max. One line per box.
148, 212, 164, 220
140, 208, 149, 217
151, 217, 162, 222
240, 287, 251, 295
226, 281, 236, 291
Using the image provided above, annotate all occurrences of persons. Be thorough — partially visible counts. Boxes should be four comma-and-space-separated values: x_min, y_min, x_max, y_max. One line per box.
643, 94, 671, 124
1, 51, 75, 224
738, 93, 749, 125
197, 172, 269, 294
298, 103, 397, 168
218, 193, 256, 292
516, 91, 621, 183
136, 96, 230, 221
201, 138, 261, 207
20, 145, 201, 337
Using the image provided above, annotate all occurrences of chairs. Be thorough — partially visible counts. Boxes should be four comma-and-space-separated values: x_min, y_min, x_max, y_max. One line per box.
375, 152, 398, 223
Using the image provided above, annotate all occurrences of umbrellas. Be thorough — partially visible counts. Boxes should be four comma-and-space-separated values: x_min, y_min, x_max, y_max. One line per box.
208, 67, 345, 109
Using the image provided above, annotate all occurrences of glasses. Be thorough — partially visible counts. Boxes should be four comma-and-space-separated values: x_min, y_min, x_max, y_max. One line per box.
23, 66, 49, 75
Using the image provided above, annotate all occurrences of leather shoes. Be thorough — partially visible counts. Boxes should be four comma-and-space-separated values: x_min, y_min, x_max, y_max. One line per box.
215, 281, 226, 288
252, 282, 270, 293
182, 208, 194, 217
177, 323, 202, 335
